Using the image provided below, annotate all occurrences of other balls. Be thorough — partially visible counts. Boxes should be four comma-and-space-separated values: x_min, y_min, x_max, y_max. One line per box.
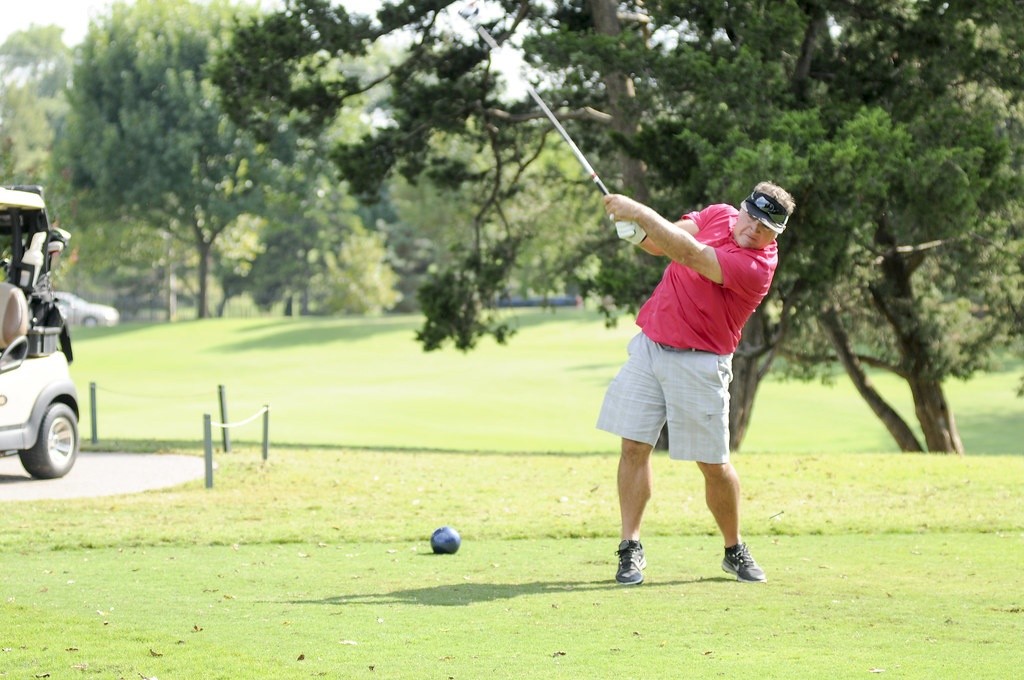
431, 526, 461, 554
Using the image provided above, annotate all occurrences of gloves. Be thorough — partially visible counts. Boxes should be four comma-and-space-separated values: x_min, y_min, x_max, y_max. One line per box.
609, 212, 648, 244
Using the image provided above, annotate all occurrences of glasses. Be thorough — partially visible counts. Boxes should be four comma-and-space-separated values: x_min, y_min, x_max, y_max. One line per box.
751, 191, 787, 224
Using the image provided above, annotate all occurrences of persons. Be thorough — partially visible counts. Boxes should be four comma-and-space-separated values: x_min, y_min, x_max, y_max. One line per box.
595, 182, 796, 584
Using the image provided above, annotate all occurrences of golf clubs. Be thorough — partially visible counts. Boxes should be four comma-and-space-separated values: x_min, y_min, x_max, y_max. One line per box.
0, 257, 11, 277
458, 5, 637, 239
51, 227, 72, 240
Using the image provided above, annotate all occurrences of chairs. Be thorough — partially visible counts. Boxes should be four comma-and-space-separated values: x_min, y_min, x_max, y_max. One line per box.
0, 283, 30, 374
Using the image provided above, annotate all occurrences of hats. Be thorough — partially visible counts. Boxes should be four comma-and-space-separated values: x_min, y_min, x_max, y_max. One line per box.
740, 191, 789, 234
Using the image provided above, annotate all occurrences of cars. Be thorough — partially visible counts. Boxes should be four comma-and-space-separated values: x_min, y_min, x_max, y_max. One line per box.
33, 291, 120, 328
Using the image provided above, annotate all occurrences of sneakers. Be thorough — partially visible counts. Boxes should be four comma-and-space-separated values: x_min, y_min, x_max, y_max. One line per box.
722, 544, 766, 584
615, 538, 646, 585
